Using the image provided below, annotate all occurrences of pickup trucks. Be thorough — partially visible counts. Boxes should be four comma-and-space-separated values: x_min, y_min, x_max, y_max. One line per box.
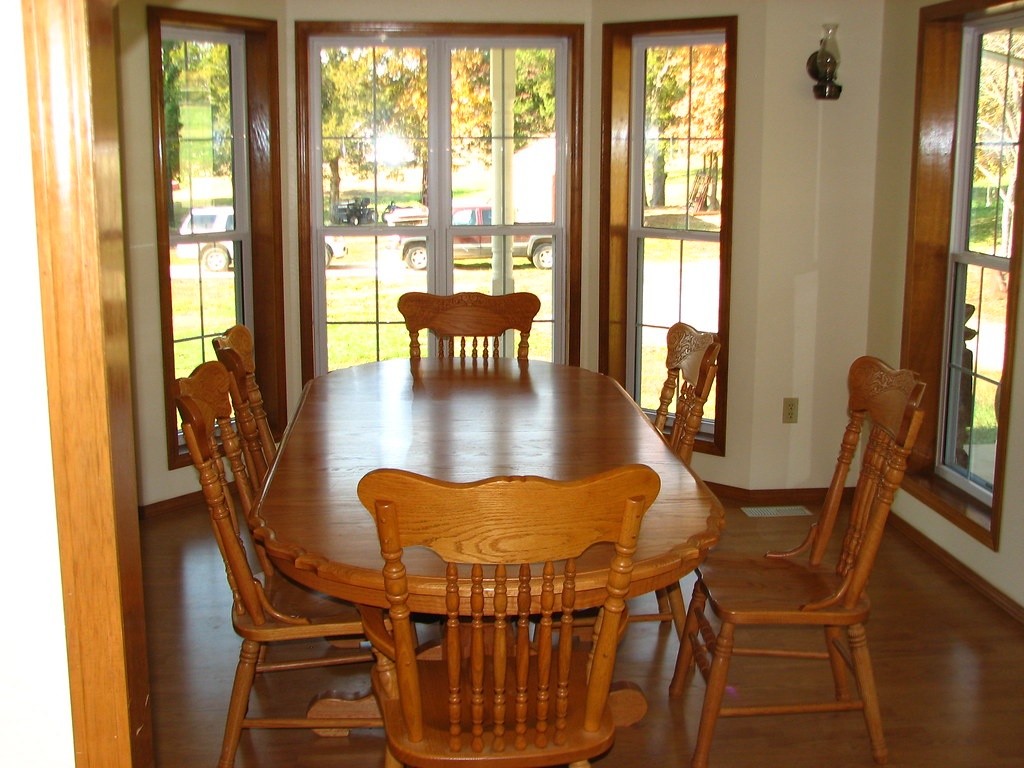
398, 205, 555, 271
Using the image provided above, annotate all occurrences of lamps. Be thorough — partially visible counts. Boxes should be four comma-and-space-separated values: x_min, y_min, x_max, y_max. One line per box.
806, 22, 843, 100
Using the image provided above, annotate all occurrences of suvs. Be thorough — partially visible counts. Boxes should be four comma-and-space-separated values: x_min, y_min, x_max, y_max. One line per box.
176, 205, 349, 272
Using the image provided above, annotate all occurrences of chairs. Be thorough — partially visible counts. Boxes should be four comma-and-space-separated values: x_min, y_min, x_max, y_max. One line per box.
173, 359, 418, 768
357, 462, 662, 767
212, 324, 319, 494
532, 322, 723, 647
397, 291, 541, 358
668, 355, 928, 768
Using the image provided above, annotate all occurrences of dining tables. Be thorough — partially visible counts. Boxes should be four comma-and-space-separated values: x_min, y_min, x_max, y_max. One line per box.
247, 358, 726, 737
